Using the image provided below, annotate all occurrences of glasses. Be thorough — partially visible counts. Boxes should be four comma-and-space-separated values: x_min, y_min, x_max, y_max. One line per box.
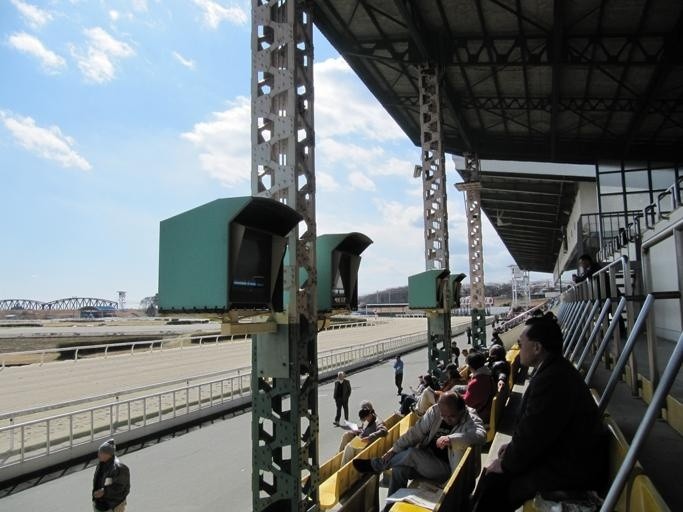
518, 339, 540, 348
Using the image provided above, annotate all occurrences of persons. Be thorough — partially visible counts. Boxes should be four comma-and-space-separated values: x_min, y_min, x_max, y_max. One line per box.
93, 439, 131, 512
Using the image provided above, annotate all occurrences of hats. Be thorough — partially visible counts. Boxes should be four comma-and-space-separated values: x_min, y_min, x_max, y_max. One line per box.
96, 438, 116, 454
358, 409, 371, 420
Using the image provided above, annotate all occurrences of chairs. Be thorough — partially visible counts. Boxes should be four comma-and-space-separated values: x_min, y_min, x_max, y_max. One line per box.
300, 339, 669, 512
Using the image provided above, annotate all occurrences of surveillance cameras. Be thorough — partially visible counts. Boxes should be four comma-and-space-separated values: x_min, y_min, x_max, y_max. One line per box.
414, 165, 422, 177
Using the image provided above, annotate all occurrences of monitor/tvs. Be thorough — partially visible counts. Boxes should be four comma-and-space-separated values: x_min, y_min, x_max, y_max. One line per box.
407, 268, 451, 308
449, 273, 467, 308
158, 196, 304, 313
282, 232, 373, 312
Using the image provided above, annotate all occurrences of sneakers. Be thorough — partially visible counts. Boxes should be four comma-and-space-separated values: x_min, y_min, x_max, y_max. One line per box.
352, 458, 382, 475
332, 421, 340, 427
